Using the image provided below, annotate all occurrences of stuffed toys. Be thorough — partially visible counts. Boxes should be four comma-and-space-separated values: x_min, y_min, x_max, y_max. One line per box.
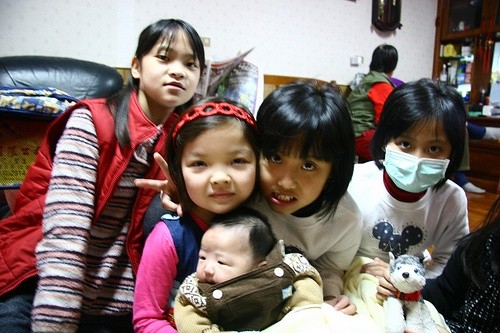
388, 252, 438, 333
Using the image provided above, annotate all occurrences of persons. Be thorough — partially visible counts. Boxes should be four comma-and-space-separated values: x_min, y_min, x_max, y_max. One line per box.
345, 44, 500, 193
346, 82, 470, 300
0, 19, 205, 333
174, 216, 323, 333
132, 97, 272, 333
421, 196, 500, 333
134, 79, 363, 333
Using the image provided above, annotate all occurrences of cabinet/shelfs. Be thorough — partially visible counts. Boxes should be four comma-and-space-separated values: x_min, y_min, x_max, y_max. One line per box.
432, 0, 500, 196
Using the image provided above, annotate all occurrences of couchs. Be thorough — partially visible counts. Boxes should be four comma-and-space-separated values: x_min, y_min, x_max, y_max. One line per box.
0, 56, 270, 218
